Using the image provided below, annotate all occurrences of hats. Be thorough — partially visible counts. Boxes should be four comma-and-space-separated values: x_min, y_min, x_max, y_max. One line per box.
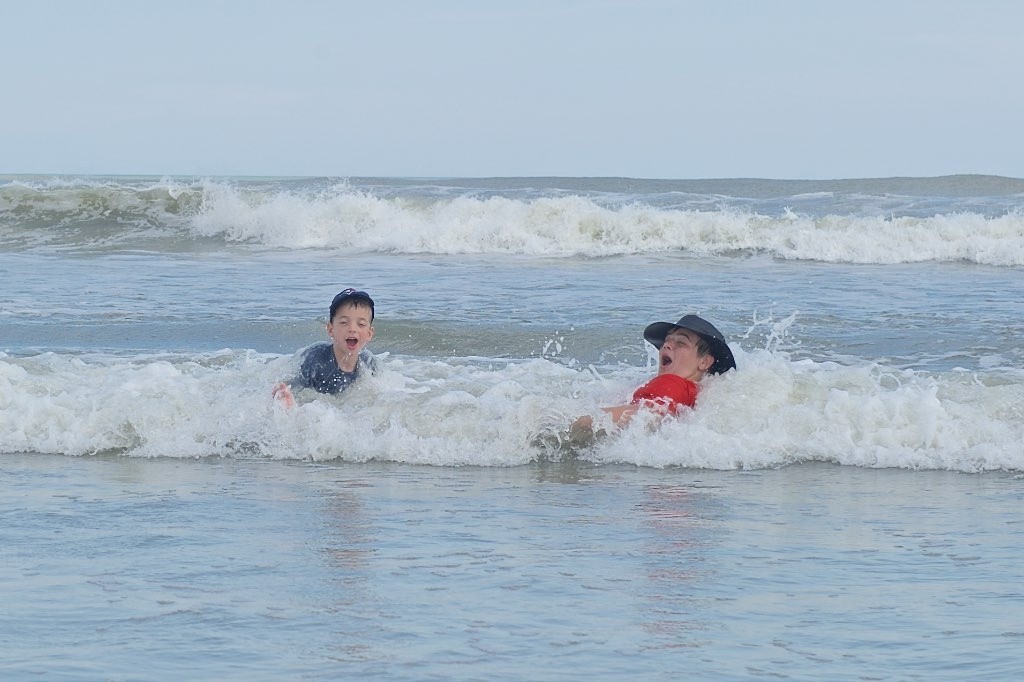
643, 315, 737, 375
329, 288, 374, 312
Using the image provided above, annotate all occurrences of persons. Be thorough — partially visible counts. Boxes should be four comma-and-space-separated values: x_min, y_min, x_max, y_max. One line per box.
274, 288, 380, 406
568, 316, 735, 428
